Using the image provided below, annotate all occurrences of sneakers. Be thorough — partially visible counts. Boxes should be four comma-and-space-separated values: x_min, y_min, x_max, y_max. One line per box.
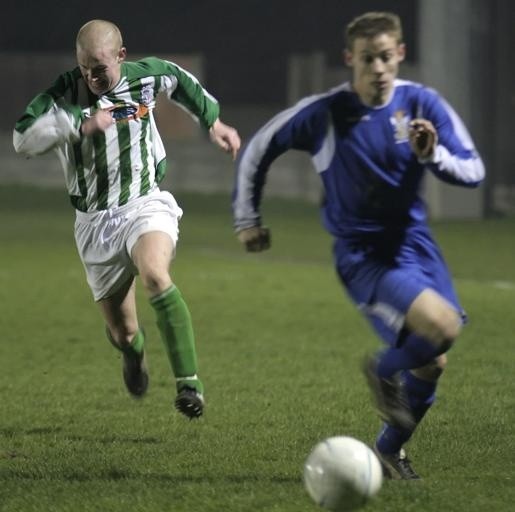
123, 350, 149, 396
371, 441, 421, 480
364, 354, 416, 433
174, 384, 204, 422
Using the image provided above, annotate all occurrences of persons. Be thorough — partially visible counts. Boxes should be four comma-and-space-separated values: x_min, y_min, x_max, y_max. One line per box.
230, 11, 487, 480
11, 18, 243, 419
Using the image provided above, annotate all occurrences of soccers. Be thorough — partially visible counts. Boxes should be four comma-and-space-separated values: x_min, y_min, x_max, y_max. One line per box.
303, 435, 382, 508
